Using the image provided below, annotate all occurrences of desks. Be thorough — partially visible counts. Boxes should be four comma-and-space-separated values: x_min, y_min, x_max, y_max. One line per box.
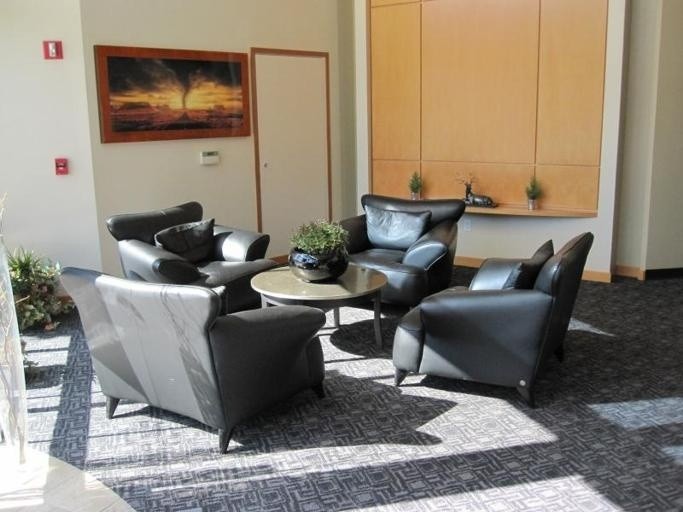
250, 265, 388, 347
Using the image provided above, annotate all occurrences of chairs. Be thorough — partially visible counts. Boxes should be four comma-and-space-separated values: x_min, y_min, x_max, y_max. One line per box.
393, 232, 594, 410
105, 201, 279, 311
336, 194, 465, 311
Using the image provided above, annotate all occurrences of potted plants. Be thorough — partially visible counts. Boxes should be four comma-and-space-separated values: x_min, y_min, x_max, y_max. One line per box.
408, 171, 423, 200
287, 215, 351, 283
525, 176, 541, 210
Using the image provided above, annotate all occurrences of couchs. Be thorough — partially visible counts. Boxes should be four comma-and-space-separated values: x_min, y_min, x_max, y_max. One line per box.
59, 267, 326, 454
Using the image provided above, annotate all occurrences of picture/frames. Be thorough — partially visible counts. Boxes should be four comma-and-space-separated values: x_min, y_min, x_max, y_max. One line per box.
93, 44, 251, 143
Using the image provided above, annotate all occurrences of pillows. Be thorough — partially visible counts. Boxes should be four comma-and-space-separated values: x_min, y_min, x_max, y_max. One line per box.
364, 204, 432, 249
154, 218, 214, 261
503, 239, 554, 289
211, 285, 229, 315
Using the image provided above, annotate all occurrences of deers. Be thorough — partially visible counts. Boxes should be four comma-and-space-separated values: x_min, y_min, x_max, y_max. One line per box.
455, 172, 496, 208
